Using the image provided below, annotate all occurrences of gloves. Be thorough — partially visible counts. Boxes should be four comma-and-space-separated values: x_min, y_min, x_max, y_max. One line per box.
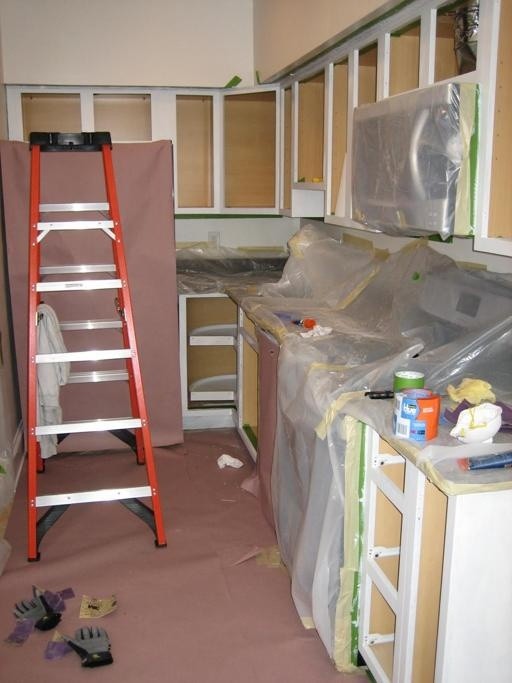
14, 596, 62, 631
61, 625, 113, 668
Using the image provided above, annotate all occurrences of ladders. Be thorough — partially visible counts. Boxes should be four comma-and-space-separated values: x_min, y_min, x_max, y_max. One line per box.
25, 131, 167, 563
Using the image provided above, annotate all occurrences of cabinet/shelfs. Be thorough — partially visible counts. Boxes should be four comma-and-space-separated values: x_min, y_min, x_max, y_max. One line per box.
179, 293, 262, 464
4, 85, 286, 217
